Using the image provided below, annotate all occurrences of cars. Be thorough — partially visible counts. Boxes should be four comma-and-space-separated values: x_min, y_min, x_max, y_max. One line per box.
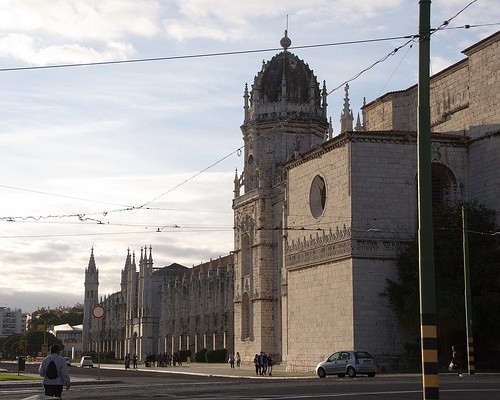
315, 350, 378, 378
64, 357, 71, 366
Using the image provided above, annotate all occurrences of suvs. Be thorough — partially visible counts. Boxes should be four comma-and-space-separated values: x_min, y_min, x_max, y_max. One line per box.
16, 356, 26, 362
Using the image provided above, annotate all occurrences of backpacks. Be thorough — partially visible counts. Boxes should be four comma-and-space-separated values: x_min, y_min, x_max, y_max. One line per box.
46, 355, 59, 380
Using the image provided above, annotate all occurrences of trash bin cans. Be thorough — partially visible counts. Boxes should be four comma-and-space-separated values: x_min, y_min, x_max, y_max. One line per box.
17, 356, 26, 371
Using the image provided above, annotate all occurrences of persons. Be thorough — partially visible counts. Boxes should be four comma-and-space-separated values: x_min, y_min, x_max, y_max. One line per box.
38, 344, 70, 397
452, 345, 463, 377
229, 352, 241, 368
124, 352, 183, 369
254, 351, 274, 377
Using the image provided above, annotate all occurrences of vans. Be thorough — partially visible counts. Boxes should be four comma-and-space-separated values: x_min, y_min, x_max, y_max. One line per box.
80, 356, 93, 368
